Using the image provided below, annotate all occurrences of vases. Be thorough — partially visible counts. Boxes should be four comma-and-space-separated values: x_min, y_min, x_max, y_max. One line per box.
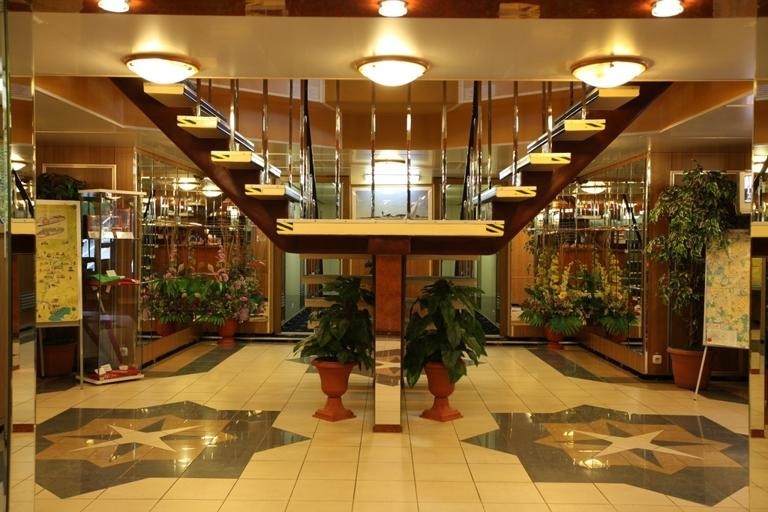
155, 316, 177, 337
543, 322, 564, 349
217, 319, 239, 344
582, 318, 601, 334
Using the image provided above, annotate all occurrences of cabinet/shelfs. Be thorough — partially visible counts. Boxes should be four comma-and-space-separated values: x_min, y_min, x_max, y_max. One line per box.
78, 188, 147, 385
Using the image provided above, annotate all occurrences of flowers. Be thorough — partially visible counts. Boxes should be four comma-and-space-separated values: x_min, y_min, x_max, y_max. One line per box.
142, 245, 194, 324
574, 249, 638, 335
194, 246, 250, 326
517, 251, 590, 334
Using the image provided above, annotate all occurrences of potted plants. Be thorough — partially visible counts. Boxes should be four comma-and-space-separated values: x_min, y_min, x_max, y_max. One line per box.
403, 278, 488, 424
644, 158, 737, 391
291, 273, 375, 421
36, 173, 88, 377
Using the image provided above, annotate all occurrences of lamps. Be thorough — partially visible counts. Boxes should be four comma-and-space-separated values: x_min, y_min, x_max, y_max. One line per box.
97, 0, 131, 15
376, 0, 409, 19
351, 54, 432, 89
120, 52, 202, 87
569, 55, 650, 89
649, 0, 686, 18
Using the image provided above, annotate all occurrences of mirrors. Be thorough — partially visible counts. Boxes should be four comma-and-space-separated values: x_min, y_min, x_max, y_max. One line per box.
137, 152, 269, 346
506, 156, 653, 355
4, 0, 36, 512
748, 0, 768, 512
